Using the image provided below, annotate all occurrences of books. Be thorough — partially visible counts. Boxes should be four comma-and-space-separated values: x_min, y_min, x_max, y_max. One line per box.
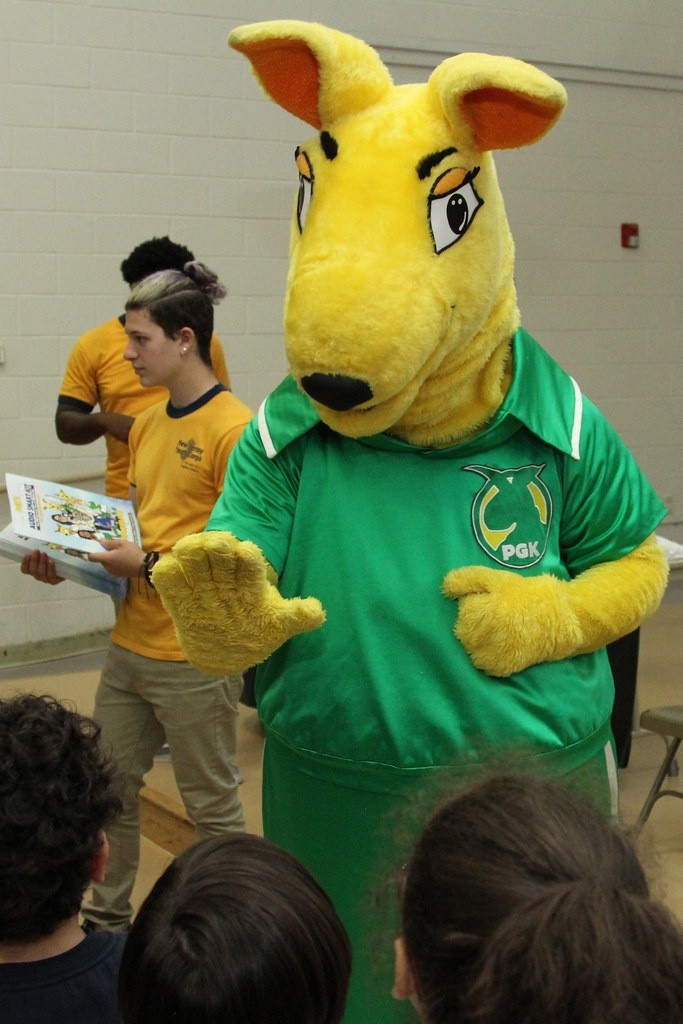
0, 473, 142, 600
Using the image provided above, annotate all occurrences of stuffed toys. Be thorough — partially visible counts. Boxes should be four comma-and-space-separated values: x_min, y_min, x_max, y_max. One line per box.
150, 19, 670, 1024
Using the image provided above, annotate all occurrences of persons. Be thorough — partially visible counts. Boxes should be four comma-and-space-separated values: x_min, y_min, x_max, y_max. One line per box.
390, 772, 683, 1024
52, 512, 72, 526
21, 236, 255, 934
0, 694, 124, 1024
78, 530, 105, 543
65, 548, 87, 561
114, 832, 353, 1024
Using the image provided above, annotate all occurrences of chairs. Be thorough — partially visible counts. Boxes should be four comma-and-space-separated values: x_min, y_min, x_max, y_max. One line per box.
624, 705, 683, 846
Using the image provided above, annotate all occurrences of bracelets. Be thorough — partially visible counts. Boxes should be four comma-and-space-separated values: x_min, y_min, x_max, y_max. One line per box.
137, 551, 160, 599
